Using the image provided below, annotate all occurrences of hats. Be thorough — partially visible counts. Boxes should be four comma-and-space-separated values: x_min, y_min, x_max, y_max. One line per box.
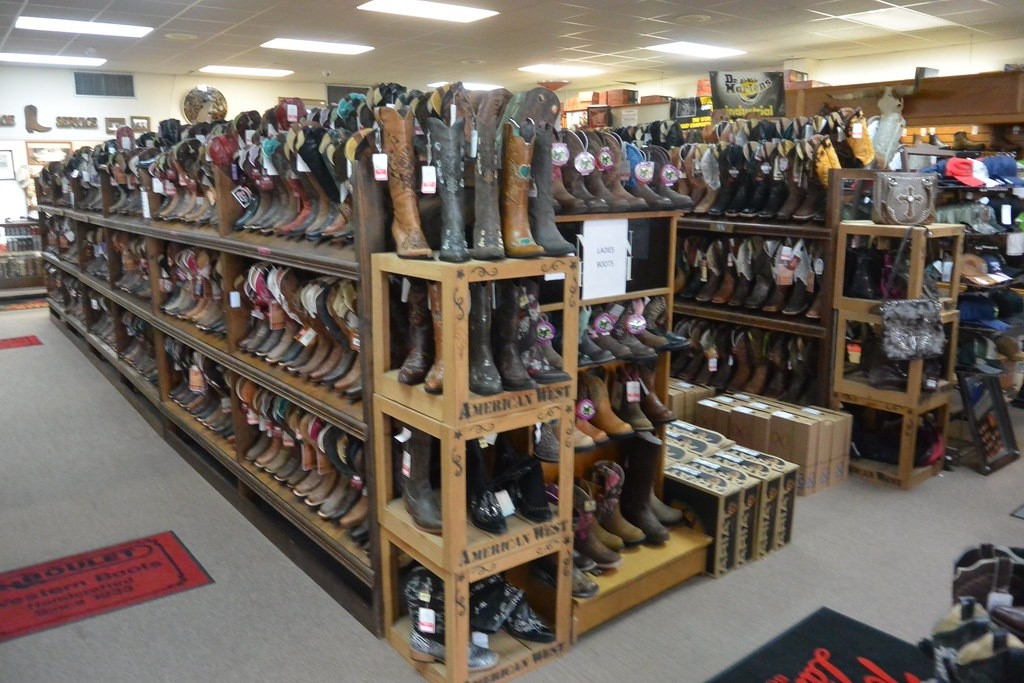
924, 153, 1024, 397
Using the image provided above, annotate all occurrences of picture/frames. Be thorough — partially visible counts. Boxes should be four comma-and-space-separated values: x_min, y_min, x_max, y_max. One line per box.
26, 142, 73, 165
0, 150, 16, 180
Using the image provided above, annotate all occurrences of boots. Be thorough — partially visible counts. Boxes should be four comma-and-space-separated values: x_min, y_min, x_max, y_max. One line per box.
34, 88, 875, 670
25, 105, 52, 133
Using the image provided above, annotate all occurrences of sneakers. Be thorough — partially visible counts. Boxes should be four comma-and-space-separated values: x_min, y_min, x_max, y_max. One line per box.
902, 126, 1024, 157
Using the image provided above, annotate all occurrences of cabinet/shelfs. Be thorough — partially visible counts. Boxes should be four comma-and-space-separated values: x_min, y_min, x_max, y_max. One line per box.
0, 221, 47, 300
30, 146, 1023, 682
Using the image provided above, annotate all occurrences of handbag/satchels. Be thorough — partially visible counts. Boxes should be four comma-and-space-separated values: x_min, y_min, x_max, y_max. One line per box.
852, 169, 945, 465
